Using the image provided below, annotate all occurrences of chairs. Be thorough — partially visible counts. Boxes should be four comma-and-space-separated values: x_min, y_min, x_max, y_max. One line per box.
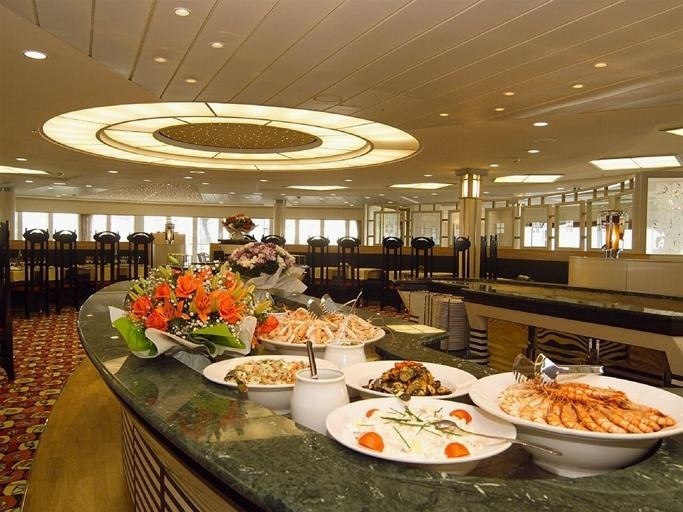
410, 233, 435, 279
124, 231, 154, 281
480, 235, 487, 279
92, 229, 121, 288
379, 235, 404, 315
335, 236, 363, 304
22, 227, 51, 321
488, 233, 497, 279
51, 228, 88, 316
304, 234, 331, 294
261, 231, 287, 250
453, 234, 472, 279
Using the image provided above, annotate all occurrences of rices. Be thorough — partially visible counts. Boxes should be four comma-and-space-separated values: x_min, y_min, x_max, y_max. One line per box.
355, 405, 494, 460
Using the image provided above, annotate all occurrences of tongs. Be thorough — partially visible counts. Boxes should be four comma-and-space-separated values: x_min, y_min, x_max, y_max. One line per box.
306, 293, 349, 319
512, 353, 605, 388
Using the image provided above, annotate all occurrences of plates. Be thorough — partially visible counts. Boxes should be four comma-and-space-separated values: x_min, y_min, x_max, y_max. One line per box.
435, 297, 471, 350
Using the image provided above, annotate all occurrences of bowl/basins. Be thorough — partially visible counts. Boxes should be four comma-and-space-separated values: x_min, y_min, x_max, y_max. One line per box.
325, 396, 520, 476
259, 301, 384, 354
470, 372, 683, 477
340, 355, 477, 399
202, 354, 339, 416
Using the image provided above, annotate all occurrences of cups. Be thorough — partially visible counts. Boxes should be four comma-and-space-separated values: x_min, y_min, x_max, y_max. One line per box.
323, 341, 366, 371
289, 369, 350, 437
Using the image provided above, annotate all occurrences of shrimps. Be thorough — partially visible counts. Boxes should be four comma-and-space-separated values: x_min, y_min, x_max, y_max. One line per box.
499, 379, 675, 434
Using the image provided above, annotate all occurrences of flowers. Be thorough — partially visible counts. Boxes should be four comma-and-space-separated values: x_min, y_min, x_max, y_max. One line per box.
220, 212, 256, 241
224, 241, 308, 310
106, 254, 278, 373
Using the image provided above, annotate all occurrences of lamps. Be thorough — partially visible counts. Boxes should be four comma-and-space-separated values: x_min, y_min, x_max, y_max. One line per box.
596, 198, 629, 261
164, 222, 178, 245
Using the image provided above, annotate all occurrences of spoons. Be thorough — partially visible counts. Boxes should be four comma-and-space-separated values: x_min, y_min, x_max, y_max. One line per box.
435, 420, 564, 458
